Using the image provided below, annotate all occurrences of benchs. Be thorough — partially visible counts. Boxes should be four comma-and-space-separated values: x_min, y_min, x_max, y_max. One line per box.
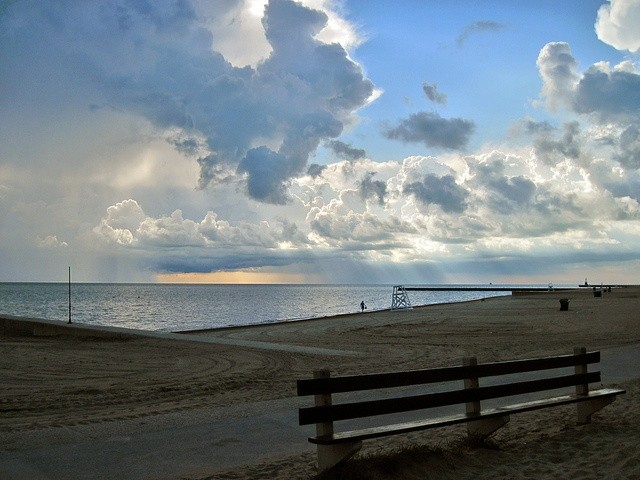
298, 346, 628, 476
